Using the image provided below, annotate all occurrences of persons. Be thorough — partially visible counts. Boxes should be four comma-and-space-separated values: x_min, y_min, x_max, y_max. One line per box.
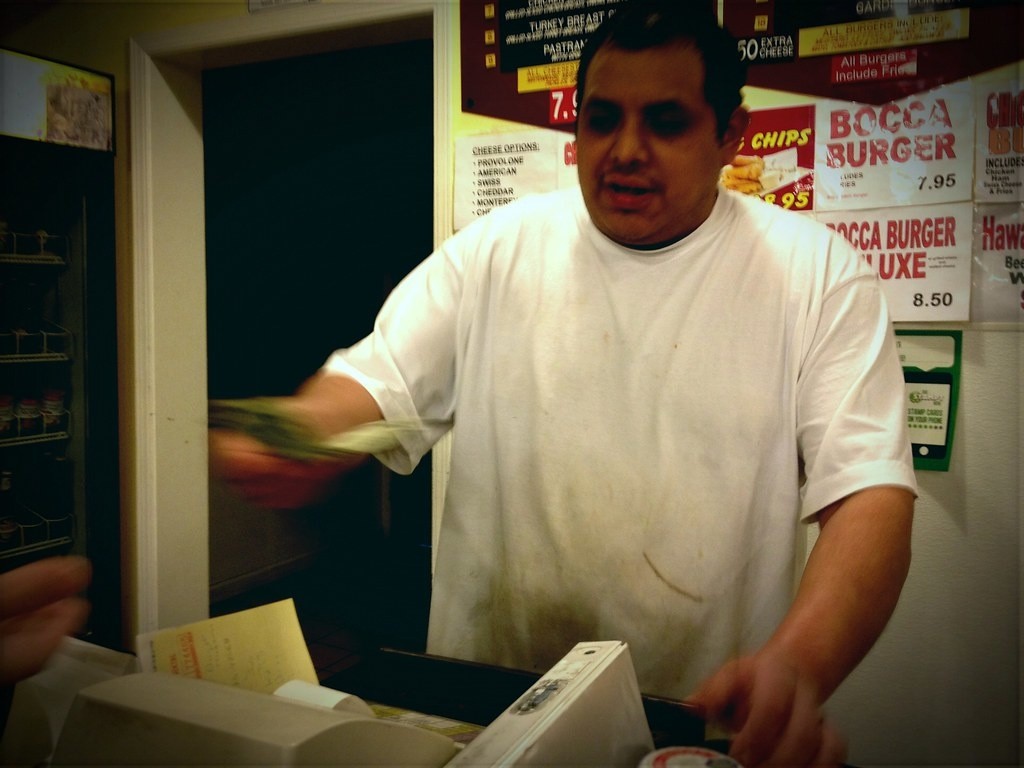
0, 553, 90, 733
207, 1, 920, 768
